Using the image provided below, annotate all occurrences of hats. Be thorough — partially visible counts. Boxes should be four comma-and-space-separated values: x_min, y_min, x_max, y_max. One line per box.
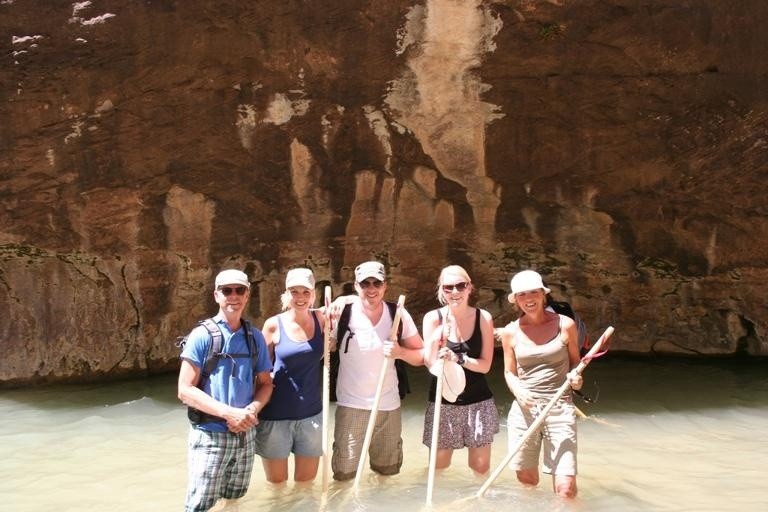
441, 360, 467, 404
215, 270, 249, 291
507, 271, 551, 304
355, 262, 386, 283
285, 269, 315, 289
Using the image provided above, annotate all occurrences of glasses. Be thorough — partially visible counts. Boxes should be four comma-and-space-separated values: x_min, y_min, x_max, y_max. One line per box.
572, 381, 600, 404
221, 287, 246, 295
359, 281, 383, 288
443, 283, 468, 292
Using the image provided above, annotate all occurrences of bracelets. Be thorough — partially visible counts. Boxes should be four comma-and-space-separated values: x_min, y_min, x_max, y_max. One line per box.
457, 353, 464, 365
245, 404, 258, 419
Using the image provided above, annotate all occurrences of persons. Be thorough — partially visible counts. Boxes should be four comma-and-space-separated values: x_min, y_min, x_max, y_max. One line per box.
501, 269, 584, 500
328, 260, 426, 481
422, 265, 501, 477
255, 266, 338, 487
493, 279, 575, 342
174, 268, 274, 512
